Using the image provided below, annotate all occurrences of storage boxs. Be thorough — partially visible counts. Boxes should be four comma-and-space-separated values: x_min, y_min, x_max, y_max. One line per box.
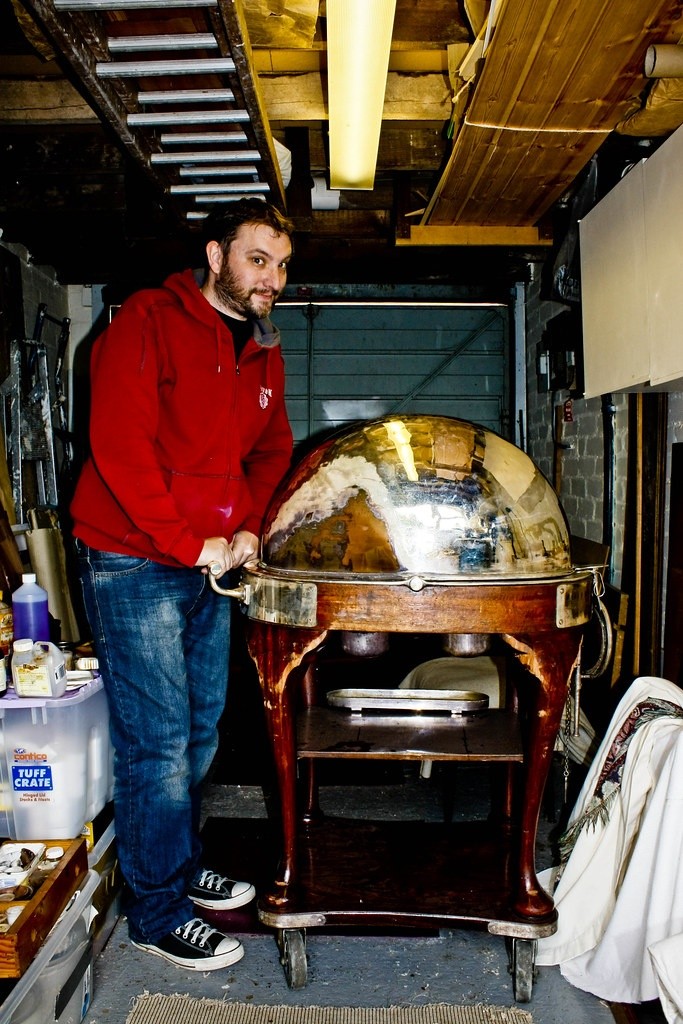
0, 672, 117, 841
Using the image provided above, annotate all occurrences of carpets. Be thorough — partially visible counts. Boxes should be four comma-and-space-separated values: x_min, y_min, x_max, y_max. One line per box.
124, 989, 535, 1024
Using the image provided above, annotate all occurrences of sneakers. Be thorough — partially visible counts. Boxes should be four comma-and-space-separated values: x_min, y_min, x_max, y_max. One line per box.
186, 867, 256, 913
130, 917, 245, 972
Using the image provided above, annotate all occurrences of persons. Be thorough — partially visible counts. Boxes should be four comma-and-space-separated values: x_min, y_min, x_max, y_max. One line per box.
73, 199, 293, 971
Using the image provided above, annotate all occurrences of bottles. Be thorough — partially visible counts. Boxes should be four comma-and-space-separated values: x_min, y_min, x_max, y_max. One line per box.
0, 590, 13, 658
11, 639, 67, 698
0, 648, 6, 698
58, 642, 72, 671
12, 574, 50, 644
